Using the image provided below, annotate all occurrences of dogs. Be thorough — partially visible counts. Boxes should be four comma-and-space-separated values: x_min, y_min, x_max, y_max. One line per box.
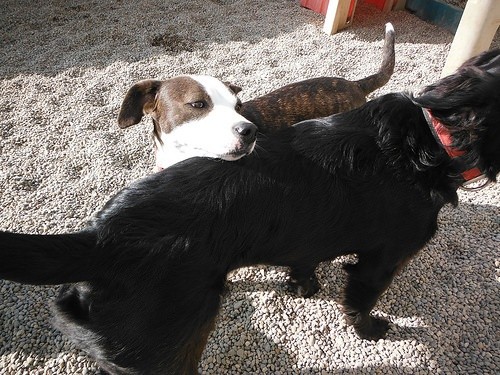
0, 46, 500, 375
118, 20, 396, 176
